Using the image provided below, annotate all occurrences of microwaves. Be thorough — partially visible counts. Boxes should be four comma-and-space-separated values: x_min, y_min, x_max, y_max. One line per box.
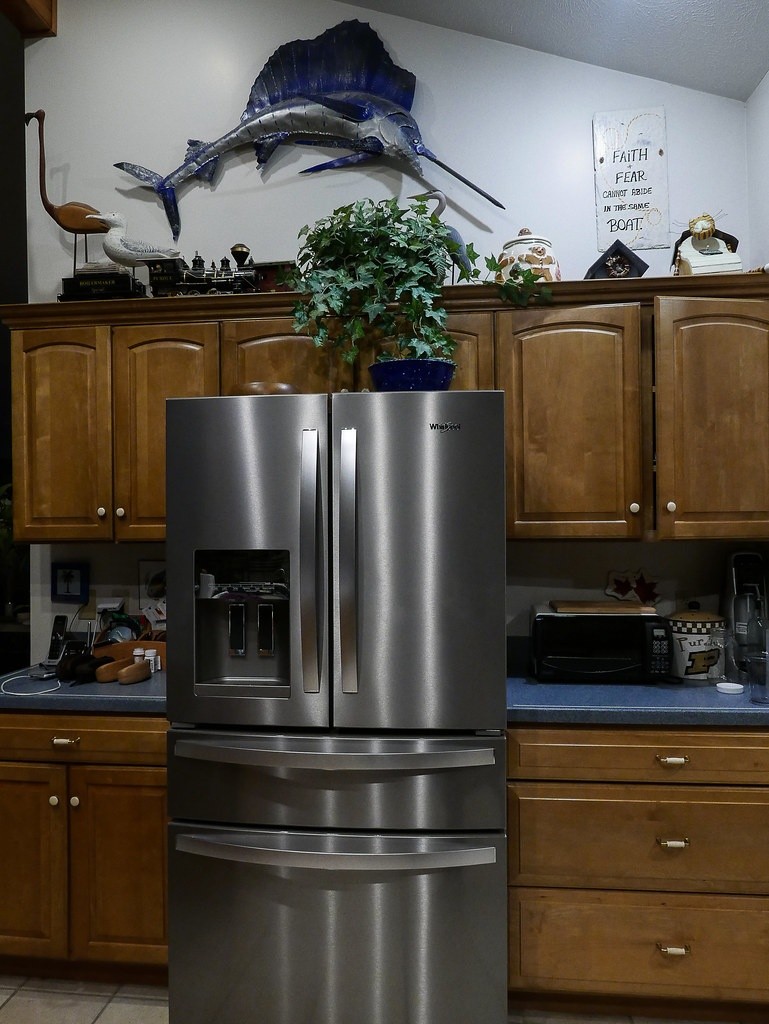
527, 604, 673, 687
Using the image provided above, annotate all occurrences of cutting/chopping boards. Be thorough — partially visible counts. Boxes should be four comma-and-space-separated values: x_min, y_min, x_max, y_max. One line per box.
549, 600, 657, 614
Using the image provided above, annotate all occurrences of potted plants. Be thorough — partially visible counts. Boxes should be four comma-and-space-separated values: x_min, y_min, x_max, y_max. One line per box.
284, 194, 560, 392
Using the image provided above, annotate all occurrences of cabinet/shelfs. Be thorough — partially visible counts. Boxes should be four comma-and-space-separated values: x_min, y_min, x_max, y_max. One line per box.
2, 708, 171, 986
0, 268, 769, 547
503, 729, 768, 1023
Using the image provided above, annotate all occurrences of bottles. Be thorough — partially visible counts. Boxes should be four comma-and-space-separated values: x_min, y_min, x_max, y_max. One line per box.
747, 596, 768, 705
705, 627, 741, 685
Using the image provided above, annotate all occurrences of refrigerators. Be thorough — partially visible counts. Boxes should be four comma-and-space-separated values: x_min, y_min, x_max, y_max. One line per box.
163, 389, 510, 1024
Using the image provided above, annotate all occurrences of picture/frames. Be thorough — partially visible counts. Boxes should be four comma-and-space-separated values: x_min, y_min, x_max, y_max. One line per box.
50, 560, 90, 604
137, 559, 167, 611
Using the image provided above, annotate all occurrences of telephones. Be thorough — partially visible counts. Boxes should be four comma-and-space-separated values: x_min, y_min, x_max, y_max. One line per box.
44, 615, 101, 667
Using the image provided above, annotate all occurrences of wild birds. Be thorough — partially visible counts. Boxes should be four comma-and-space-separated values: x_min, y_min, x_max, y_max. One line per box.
406, 190, 472, 286
24, 108, 110, 278
84, 212, 180, 278
